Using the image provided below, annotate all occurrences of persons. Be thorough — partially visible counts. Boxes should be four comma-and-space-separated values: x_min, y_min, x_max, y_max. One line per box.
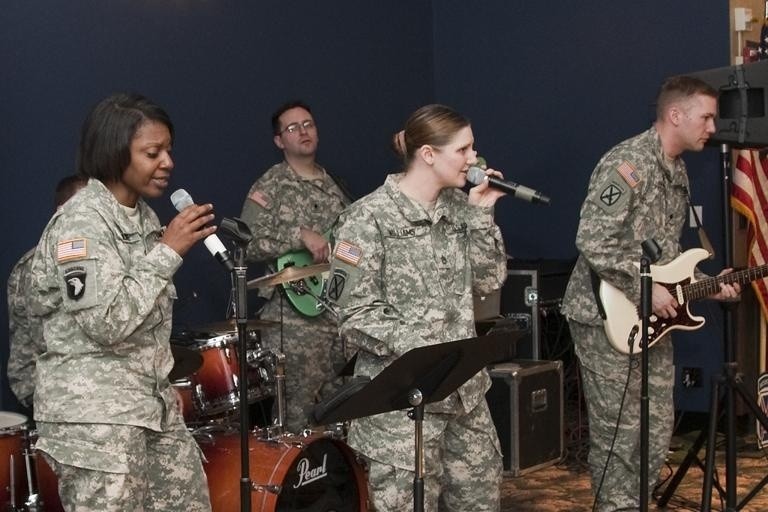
240, 102, 356, 436
316, 104, 511, 511
558, 74, 745, 512
6, 172, 90, 411
31, 91, 218, 511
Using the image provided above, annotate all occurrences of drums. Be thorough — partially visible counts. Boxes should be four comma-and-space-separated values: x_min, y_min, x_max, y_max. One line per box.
168, 380, 204, 437
31, 426, 64, 512
0, 411, 31, 512
194, 422, 370, 510
189, 329, 278, 421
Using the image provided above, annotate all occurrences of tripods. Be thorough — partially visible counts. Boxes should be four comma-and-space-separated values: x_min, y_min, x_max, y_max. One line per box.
656, 145, 768, 512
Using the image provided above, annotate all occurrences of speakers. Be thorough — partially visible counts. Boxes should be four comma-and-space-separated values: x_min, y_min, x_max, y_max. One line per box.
678, 59, 768, 152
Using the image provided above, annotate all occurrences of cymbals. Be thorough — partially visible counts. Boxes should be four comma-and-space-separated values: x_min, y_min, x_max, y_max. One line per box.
184, 316, 280, 336
164, 343, 205, 383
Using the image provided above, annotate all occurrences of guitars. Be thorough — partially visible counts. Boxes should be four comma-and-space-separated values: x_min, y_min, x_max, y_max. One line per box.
593, 248, 768, 357
274, 221, 334, 318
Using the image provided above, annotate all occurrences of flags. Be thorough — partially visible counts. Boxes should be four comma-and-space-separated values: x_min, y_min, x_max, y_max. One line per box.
729, 46, 767, 326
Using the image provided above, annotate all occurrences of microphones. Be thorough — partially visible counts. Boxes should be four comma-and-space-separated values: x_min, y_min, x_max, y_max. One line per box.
171, 189, 236, 272
466, 167, 552, 207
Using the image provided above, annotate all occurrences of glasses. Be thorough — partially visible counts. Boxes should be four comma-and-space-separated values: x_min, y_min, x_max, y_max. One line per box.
279, 120, 316, 137
